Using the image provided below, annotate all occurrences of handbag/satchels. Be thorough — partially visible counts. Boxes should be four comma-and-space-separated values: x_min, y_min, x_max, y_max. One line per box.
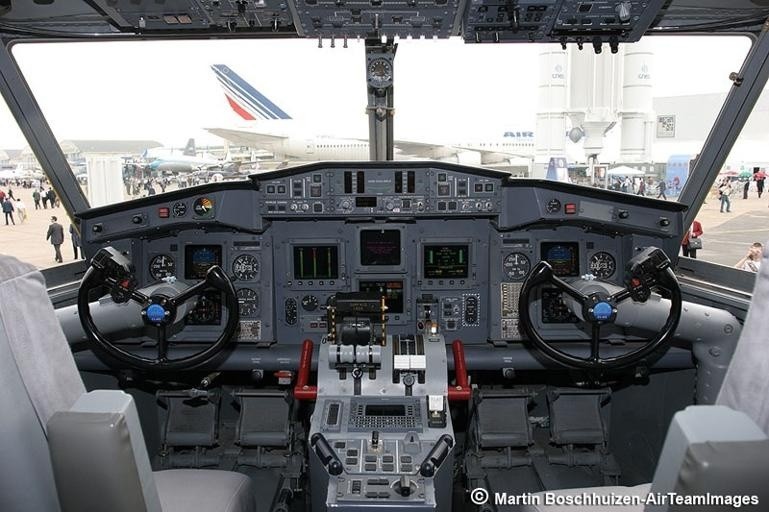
686, 234, 702, 250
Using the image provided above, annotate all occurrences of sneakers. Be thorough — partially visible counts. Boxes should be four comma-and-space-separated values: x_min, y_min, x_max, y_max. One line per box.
720, 209, 732, 213
54, 254, 87, 264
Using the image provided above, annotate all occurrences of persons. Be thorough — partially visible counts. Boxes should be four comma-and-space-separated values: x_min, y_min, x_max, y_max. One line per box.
735, 242, 764, 274
1, 156, 249, 225
46, 215, 64, 263
69, 223, 85, 260
569, 166, 769, 213
681, 221, 703, 259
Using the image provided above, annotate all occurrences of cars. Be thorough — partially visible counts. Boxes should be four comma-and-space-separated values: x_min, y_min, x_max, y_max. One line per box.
196, 164, 232, 183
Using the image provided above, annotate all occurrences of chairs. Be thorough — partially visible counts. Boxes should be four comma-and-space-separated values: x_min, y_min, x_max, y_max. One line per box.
522, 234, 769, 512
0, 251, 254, 512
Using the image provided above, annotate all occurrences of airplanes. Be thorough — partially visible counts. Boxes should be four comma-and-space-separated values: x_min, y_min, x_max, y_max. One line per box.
141, 138, 232, 171
204, 63, 535, 167
